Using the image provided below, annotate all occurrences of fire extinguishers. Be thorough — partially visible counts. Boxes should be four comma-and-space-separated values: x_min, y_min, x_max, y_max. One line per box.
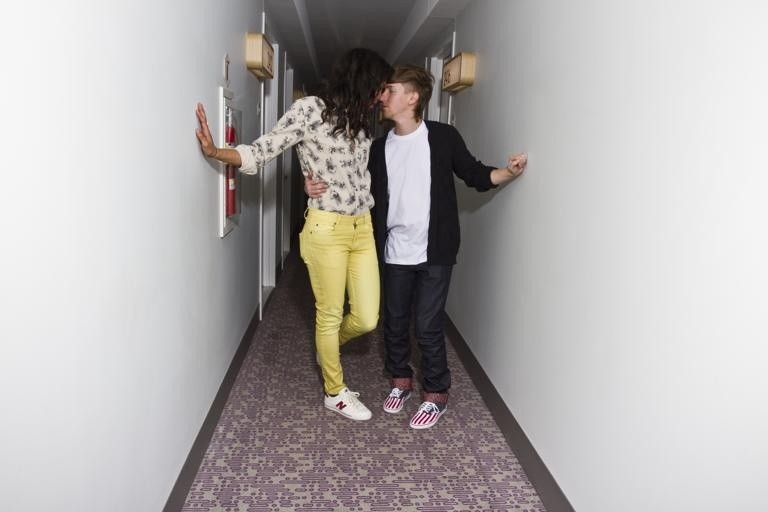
226, 108, 239, 217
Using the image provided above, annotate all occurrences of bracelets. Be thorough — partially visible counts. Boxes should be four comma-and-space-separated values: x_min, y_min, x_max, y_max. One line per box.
212, 148, 218, 158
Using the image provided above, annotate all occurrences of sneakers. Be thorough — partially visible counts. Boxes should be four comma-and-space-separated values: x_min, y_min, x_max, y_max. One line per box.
323, 387, 372, 421
383, 388, 413, 413
409, 400, 448, 429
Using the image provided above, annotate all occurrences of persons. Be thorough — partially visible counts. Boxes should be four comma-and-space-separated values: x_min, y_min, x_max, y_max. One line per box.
301, 60, 528, 430
195, 44, 394, 423
